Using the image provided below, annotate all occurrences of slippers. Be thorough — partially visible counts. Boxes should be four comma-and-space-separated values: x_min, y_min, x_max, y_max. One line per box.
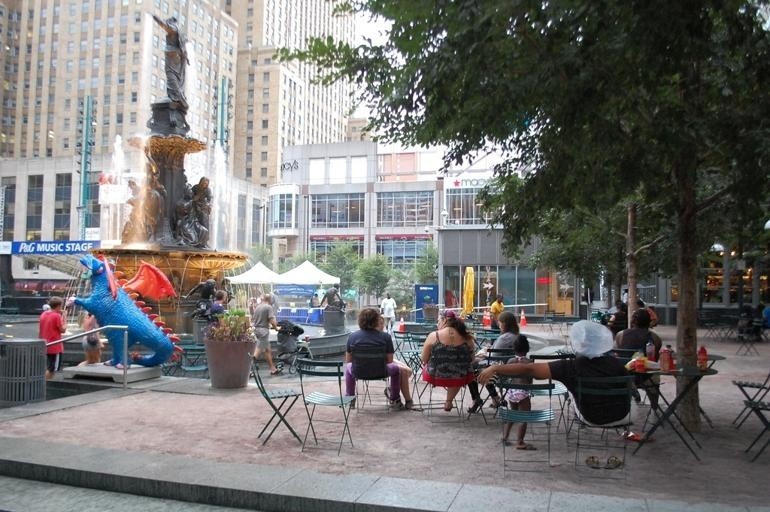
501, 439, 515, 446
270, 371, 281, 375
586, 455, 601, 469
606, 456, 622, 471
515, 442, 537, 451
251, 372, 257, 378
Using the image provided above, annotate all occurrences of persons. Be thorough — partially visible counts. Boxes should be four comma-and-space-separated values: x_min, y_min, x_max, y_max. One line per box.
490, 294, 505, 345
318, 283, 347, 309
309, 292, 319, 307
78, 310, 104, 369
249, 294, 283, 377
150, 17, 192, 103
181, 278, 218, 319
615, 309, 663, 409
191, 177, 213, 238
478, 320, 636, 427
501, 334, 539, 450
608, 299, 623, 316
606, 303, 629, 339
38, 295, 67, 379
468, 312, 521, 412
756, 297, 769, 341
175, 187, 205, 248
636, 300, 658, 328
374, 318, 414, 408
209, 290, 228, 319
622, 289, 629, 305
343, 308, 404, 411
381, 292, 397, 333
421, 310, 476, 410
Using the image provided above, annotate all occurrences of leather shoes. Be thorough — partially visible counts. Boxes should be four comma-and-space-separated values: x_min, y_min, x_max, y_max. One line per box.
634, 396, 641, 403
652, 402, 657, 409
490, 400, 508, 408
468, 399, 484, 413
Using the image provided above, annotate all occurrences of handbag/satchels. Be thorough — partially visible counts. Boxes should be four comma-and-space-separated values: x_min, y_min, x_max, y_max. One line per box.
475, 348, 488, 357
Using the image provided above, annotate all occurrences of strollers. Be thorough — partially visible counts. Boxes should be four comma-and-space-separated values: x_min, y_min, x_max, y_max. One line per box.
271, 320, 314, 375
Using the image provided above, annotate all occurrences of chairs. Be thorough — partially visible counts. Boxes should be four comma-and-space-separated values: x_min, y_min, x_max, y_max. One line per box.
530, 353, 569, 435
247, 352, 304, 446
537, 310, 555, 333
548, 312, 565, 335
613, 348, 646, 366
425, 349, 471, 426
568, 376, 635, 479
733, 372, 770, 428
351, 344, 394, 414
467, 348, 514, 425
394, 331, 413, 362
743, 399, 769, 461
296, 358, 357, 455
498, 373, 555, 478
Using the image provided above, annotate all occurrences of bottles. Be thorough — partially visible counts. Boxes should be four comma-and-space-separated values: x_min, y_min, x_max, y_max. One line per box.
698, 346, 708, 371
666, 345, 673, 368
400, 318, 405, 331
635, 356, 644, 372
625, 355, 638, 371
660, 349, 669, 371
645, 339, 655, 361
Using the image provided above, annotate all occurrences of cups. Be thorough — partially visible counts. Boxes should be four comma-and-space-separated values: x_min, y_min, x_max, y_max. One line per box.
634, 360, 646, 373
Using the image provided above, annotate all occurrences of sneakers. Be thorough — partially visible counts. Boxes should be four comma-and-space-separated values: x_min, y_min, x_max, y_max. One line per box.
339, 399, 355, 409
387, 330, 388, 332
391, 330, 393, 332
390, 399, 405, 410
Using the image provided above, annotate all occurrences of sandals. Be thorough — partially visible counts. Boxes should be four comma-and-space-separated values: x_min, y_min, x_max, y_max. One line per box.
406, 402, 416, 409
443, 400, 453, 412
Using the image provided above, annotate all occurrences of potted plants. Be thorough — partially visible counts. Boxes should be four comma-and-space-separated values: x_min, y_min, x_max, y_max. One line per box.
201, 309, 256, 389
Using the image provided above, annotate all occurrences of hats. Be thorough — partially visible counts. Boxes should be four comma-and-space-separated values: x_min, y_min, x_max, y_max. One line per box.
440, 310, 458, 319
495, 293, 503, 298
570, 320, 614, 358
